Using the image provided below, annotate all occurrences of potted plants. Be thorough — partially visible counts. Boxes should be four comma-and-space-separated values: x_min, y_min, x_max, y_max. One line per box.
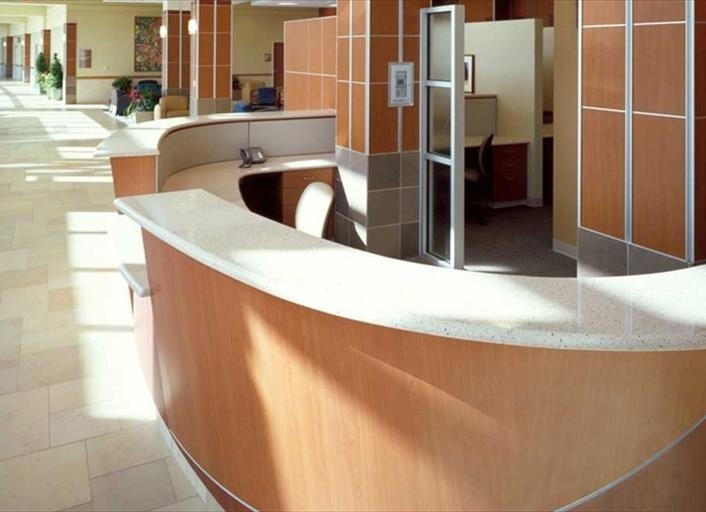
34, 52, 62, 100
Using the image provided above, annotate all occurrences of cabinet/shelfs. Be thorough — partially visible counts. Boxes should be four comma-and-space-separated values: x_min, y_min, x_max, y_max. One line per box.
280, 168, 336, 230
490, 143, 528, 204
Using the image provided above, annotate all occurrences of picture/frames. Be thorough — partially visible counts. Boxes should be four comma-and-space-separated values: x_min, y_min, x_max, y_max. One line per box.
133, 16, 163, 72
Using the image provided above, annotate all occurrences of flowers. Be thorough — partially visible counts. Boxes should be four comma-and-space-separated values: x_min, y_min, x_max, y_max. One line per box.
122, 88, 154, 113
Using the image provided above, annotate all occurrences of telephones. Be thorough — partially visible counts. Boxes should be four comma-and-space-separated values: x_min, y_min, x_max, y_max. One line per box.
240, 147, 265, 164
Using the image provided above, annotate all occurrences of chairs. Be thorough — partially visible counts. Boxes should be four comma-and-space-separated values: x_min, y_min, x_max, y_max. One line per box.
154, 96, 190, 121
294, 180, 335, 239
243, 88, 276, 112
437, 131, 497, 227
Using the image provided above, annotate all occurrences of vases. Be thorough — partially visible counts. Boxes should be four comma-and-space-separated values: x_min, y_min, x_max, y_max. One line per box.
127, 110, 153, 125
